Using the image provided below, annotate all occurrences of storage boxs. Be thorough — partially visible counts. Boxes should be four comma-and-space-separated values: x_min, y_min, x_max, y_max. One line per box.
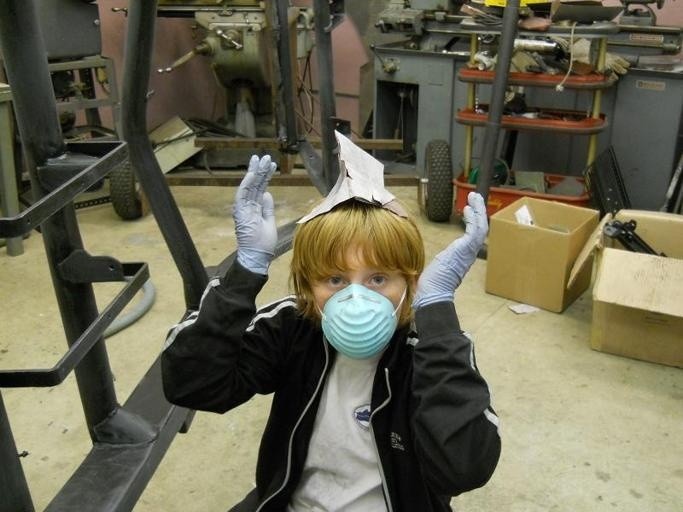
567, 209, 683, 369
485, 196, 600, 313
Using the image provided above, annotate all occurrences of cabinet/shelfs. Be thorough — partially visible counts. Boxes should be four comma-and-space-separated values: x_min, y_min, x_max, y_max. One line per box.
453, 18, 619, 219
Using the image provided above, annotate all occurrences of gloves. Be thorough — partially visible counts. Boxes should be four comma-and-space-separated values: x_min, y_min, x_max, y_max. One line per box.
408, 190, 490, 314
229, 153, 280, 280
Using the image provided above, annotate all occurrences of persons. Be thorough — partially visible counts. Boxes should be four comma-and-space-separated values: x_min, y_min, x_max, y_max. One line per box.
159, 152, 502, 512
